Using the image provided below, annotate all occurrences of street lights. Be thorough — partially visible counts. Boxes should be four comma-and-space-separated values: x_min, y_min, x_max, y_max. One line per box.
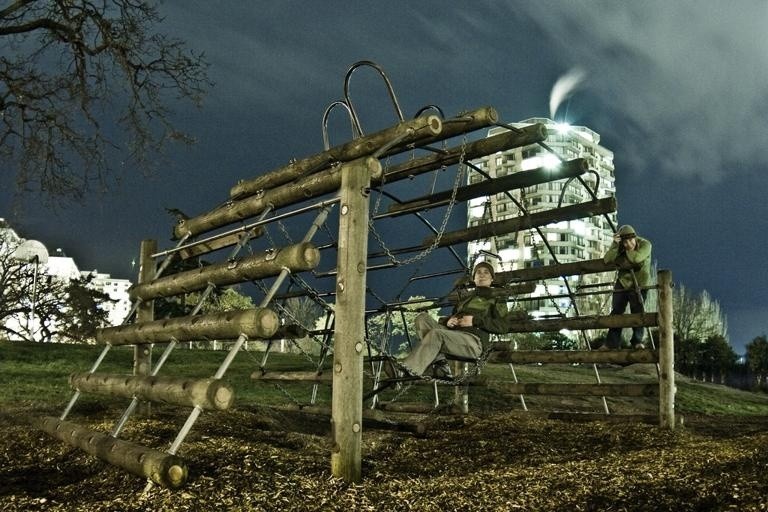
27, 254, 40, 342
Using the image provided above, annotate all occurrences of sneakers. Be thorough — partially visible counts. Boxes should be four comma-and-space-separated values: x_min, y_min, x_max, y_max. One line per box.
431, 364, 454, 381
382, 355, 403, 390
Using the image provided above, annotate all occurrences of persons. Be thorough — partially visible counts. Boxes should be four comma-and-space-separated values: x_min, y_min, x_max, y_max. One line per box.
597, 224, 652, 352
385, 262, 509, 395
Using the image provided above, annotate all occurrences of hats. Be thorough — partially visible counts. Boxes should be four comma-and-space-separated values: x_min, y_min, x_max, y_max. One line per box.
618, 225, 637, 237
473, 262, 494, 279
